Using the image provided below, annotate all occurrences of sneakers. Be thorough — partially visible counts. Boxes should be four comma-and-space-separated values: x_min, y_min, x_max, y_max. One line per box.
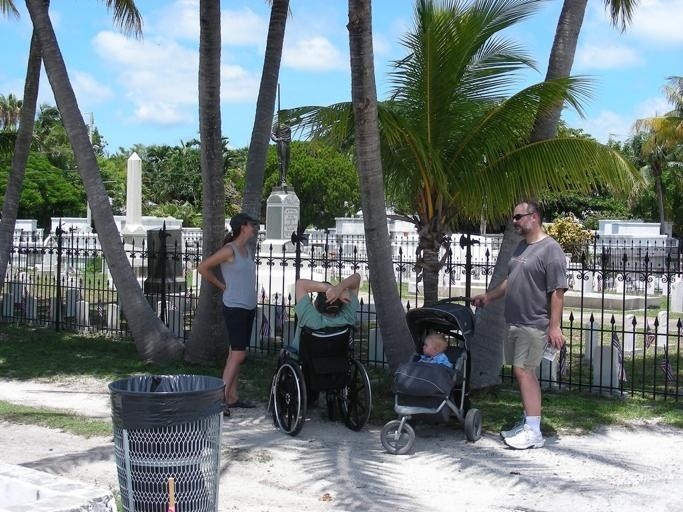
224, 398, 256, 417
499, 421, 545, 450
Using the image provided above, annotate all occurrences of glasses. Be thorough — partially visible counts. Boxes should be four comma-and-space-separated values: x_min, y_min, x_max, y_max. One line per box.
512, 213, 535, 219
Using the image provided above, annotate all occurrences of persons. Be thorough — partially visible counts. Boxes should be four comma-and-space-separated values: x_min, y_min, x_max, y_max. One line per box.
414, 330, 454, 371
194, 211, 261, 418
285, 271, 362, 409
468, 200, 569, 452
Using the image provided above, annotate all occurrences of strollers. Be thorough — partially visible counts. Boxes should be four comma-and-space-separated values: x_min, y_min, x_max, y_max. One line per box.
380, 296, 482, 455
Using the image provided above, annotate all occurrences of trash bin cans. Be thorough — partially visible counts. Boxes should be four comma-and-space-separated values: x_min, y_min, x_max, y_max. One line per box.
107, 373, 226, 505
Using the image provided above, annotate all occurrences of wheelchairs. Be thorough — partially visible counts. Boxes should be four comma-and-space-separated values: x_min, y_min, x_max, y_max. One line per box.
265, 324, 372, 437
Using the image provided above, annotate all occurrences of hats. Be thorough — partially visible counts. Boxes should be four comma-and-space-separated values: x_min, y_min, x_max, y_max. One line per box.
229, 212, 260, 228
318, 281, 343, 312
